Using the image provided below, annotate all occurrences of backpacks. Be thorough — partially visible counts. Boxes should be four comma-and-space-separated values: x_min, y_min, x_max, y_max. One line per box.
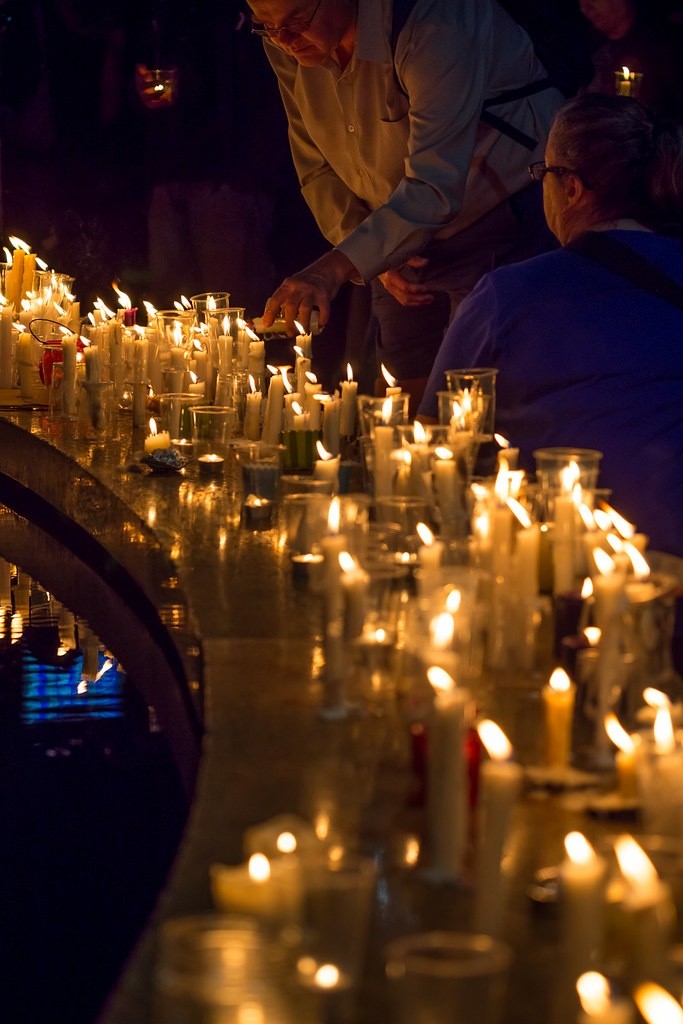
390, 0, 596, 151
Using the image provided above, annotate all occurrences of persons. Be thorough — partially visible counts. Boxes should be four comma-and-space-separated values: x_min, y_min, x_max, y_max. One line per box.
135, 0, 285, 317
580, 0, 683, 113
415, 94, 683, 679
247, 0, 564, 428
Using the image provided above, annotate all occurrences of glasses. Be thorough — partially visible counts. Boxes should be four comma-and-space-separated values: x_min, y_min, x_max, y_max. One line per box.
528, 161, 593, 191
251, 0, 322, 38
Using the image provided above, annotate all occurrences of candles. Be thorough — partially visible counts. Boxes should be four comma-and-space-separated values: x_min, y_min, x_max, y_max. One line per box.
0, 556, 129, 694
615, 66, 644, 98
0, 235, 683, 1024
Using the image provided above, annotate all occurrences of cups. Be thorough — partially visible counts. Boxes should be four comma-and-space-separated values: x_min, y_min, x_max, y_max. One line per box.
614, 71, 643, 96
142, 68, 174, 101
0, 261, 683, 1024
